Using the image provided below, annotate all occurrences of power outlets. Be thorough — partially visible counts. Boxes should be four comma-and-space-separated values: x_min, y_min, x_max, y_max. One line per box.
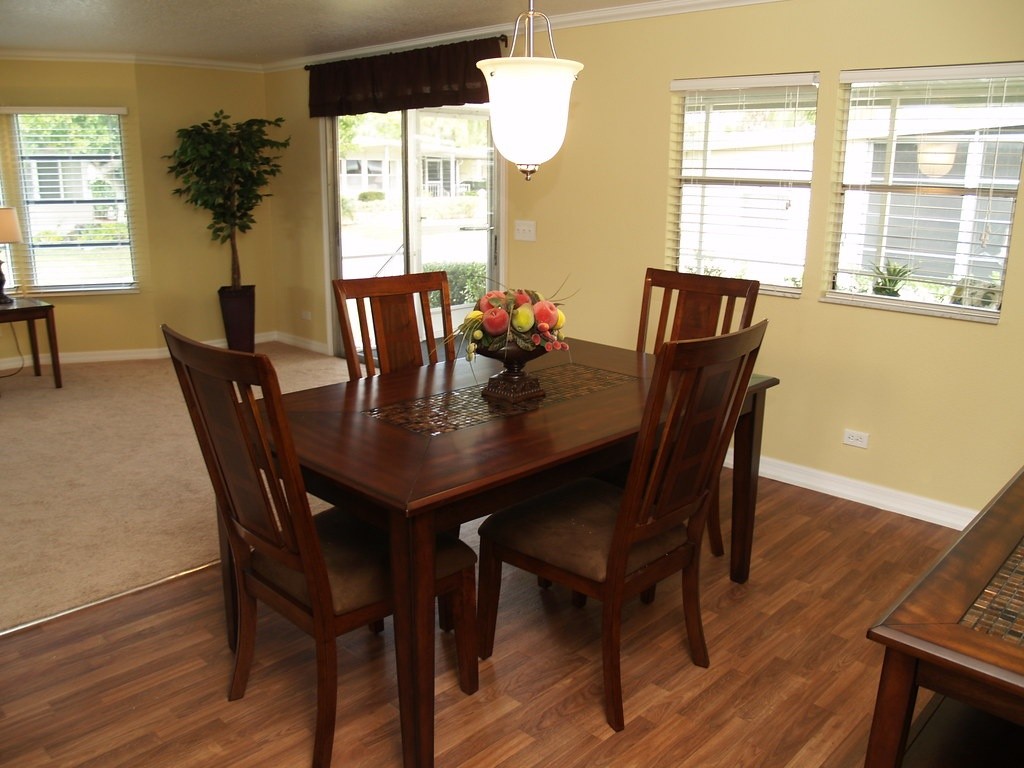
842, 428, 870, 450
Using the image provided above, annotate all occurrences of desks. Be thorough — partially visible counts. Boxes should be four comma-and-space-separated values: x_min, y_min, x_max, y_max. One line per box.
0, 298, 62, 389
197, 339, 779, 768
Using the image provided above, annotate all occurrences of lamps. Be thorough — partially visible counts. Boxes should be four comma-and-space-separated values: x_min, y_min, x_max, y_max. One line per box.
476, 0, 584, 180
0, 207, 24, 304
914, 138, 959, 178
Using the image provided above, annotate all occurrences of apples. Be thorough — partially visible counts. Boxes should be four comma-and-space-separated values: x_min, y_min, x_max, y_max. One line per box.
480, 290, 558, 337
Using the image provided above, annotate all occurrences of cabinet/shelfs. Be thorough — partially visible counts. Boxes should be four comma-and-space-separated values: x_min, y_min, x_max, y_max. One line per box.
860, 465, 1024, 768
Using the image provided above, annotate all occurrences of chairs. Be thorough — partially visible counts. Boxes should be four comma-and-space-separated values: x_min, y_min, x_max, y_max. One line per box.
578, 268, 761, 605
161, 320, 481, 768
332, 270, 454, 635
471, 321, 769, 737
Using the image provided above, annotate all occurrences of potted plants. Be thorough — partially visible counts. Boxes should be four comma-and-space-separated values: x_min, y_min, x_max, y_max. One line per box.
870, 261, 921, 298
168, 111, 294, 353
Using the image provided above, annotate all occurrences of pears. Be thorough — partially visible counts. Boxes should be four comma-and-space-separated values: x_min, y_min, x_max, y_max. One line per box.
512, 302, 534, 332
552, 309, 565, 330
466, 310, 483, 322
